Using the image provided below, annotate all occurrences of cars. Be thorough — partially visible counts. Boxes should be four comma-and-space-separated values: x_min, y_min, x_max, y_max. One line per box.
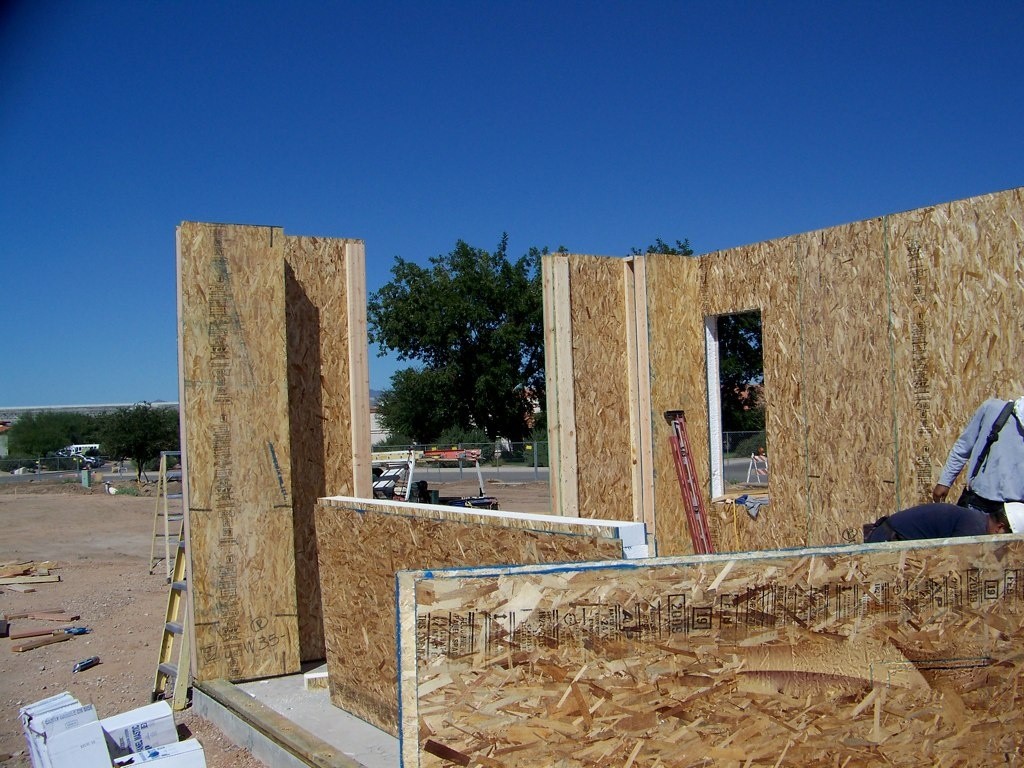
54, 449, 105, 468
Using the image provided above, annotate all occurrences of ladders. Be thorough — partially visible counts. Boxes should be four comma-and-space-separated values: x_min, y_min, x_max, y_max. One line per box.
149, 451, 187, 586
151, 519, 194, 709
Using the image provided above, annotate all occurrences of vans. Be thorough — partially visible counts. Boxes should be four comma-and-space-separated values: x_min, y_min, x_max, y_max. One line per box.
66, 444, 101, 456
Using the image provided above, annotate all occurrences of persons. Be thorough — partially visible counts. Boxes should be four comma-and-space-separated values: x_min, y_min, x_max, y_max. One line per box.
931, 396, 1024, 518
863, 501, 1024, 546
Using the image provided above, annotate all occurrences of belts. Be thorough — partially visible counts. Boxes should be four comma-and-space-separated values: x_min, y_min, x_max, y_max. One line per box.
881, 521, 893, 535
